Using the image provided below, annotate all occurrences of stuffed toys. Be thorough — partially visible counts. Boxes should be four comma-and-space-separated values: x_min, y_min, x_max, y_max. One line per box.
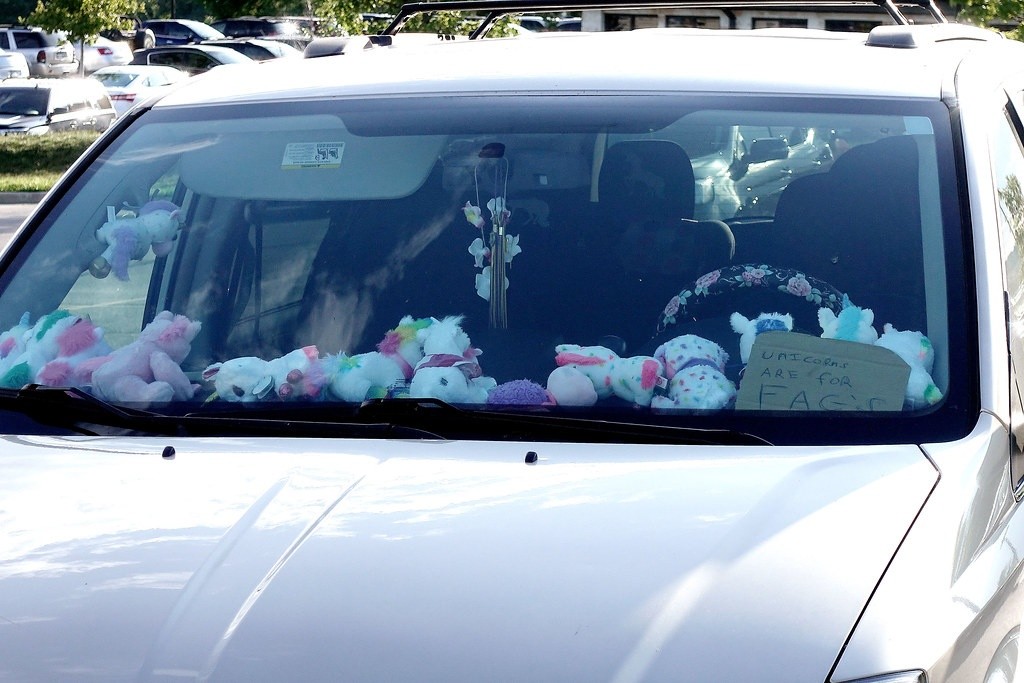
817, 305, 878, 346
875, 324, 942, 410
90, 199, 183, 280
0, 310, 113, 394
731, 311, 793, 386
650, 334, 736, 416
486, 380, 550, 404
296, 317, 425, 403
204, 346, 319, 403
411, 315, 489, 407
76, 310, 202, 408
546, 343, 663, 408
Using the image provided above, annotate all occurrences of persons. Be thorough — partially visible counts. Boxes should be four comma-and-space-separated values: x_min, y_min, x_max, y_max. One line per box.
134, 25, 146, 49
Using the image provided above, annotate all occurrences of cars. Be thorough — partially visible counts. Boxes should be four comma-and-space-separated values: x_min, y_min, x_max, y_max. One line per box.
60, 29, 134, 72
0, 48, 29, 82
90, 66, 190, 119
230, 39, 300, 64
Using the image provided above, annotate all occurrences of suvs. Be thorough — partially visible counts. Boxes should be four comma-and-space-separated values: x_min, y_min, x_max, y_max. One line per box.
0, 24, 80, 78
211, 18, 302, 45
100, 17, 156, 53
0, 21, 1024, 683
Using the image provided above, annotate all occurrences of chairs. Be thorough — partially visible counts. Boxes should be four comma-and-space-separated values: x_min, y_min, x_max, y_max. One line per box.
601, 136, 962, 333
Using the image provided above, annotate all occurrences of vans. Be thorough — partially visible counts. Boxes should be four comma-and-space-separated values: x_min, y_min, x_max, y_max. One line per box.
141, 20, 224, 48
284, 17, 350, 40
129, 47, 254, 76
0, 79, 117, 135
